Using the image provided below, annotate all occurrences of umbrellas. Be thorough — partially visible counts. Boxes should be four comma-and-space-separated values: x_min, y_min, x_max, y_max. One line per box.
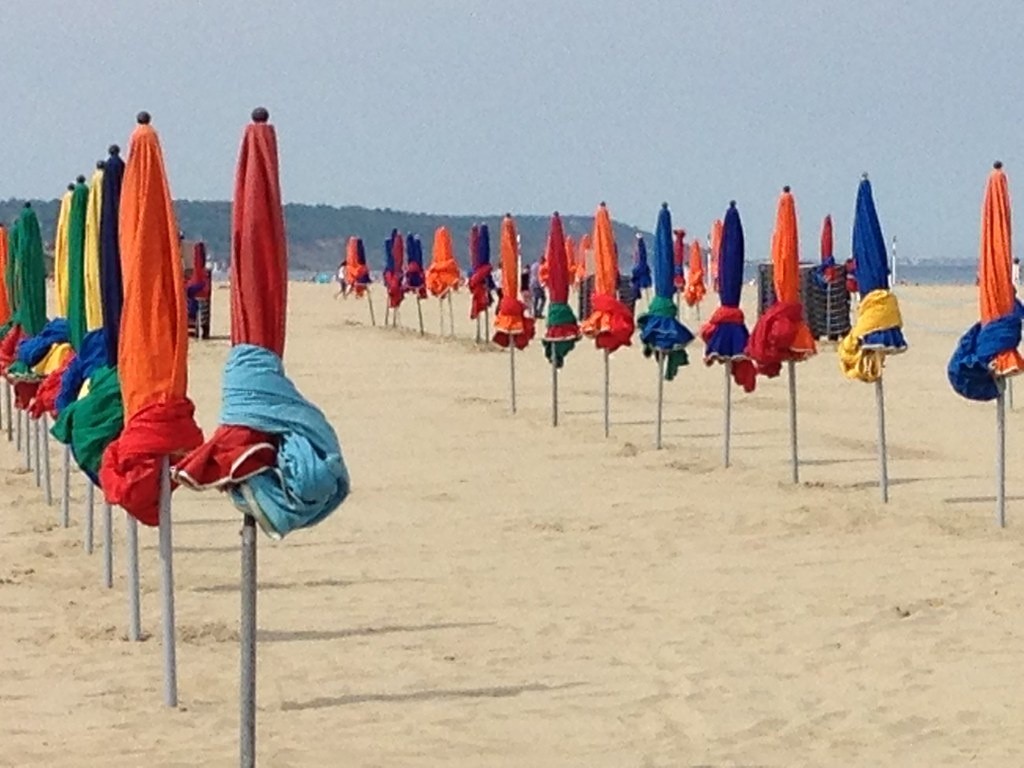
347, 235, 377, 326
0, 107, 350, 768
542, 212, 582, 428
589, 159, 1024, 537
492, 213, 537, 412
383, 220, 492, 345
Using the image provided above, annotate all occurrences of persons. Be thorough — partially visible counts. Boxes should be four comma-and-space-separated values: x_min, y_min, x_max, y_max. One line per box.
1011, 258, 1022, 284
333, 257, 363, 303
494, 255, 546, 319
